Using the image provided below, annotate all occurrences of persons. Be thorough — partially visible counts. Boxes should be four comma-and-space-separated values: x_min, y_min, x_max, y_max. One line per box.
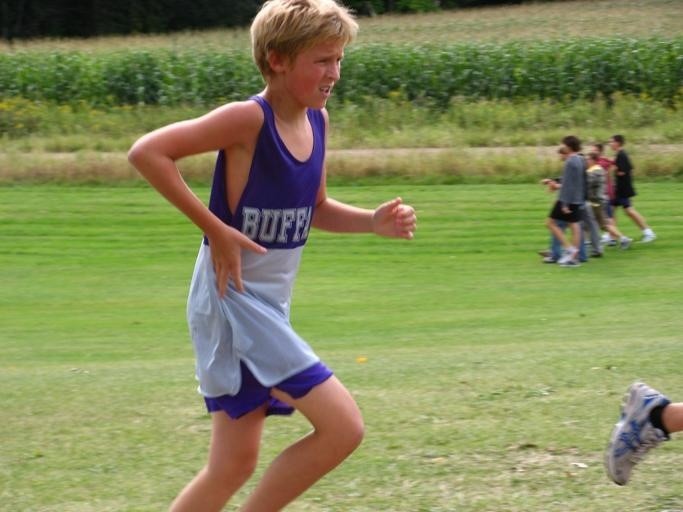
125, 0, 419, 512
604, 381, 682, 486
536, 135, 658, 268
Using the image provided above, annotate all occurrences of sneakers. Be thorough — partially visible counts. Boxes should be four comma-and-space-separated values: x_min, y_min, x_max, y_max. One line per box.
604, 380, 669, 487
537, 229, 657, 267
